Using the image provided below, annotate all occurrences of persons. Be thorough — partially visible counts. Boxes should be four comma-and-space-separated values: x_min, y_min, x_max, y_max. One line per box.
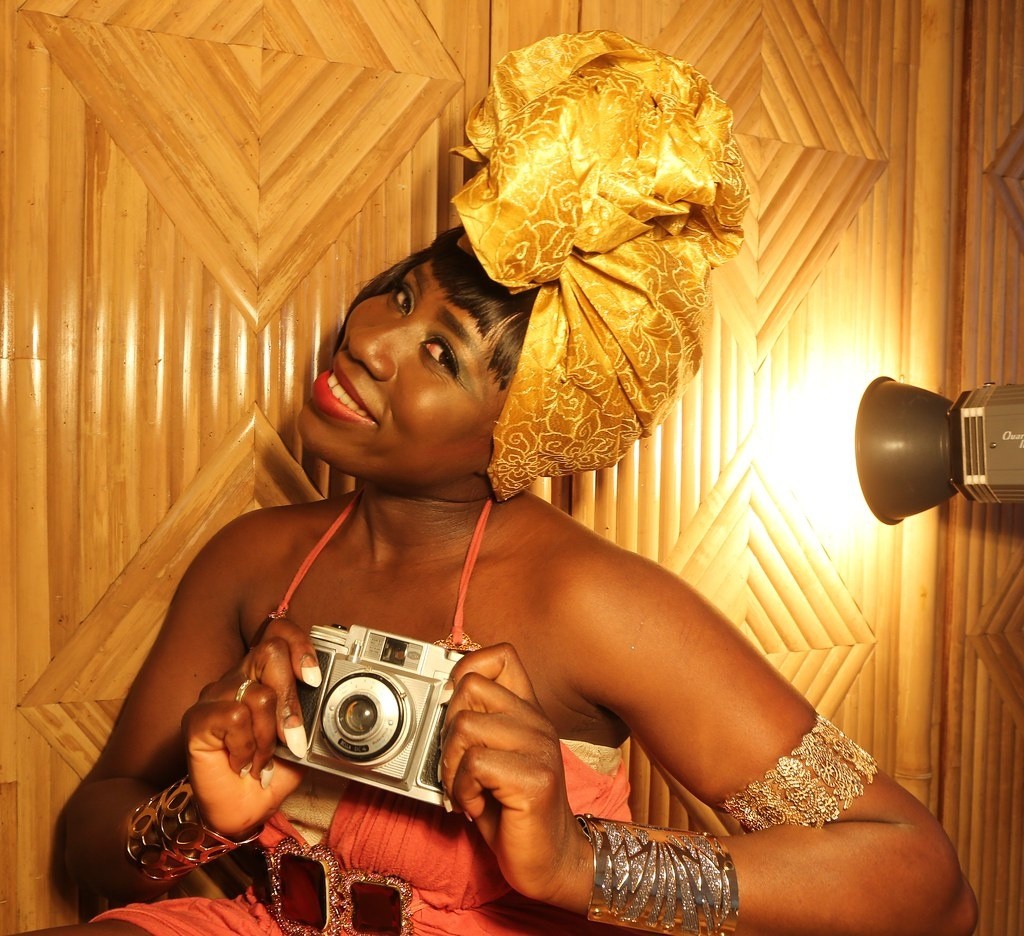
3, 210, 985, 936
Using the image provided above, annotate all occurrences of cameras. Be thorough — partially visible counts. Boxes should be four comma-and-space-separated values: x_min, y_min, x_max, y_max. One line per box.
274, 624, 472, 810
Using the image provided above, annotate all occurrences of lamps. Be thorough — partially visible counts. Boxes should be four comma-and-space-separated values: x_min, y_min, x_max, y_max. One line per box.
854, 374, 1024, 526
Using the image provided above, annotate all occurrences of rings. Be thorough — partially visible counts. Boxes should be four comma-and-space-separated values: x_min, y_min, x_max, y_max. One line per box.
231, 675, 266, 705
